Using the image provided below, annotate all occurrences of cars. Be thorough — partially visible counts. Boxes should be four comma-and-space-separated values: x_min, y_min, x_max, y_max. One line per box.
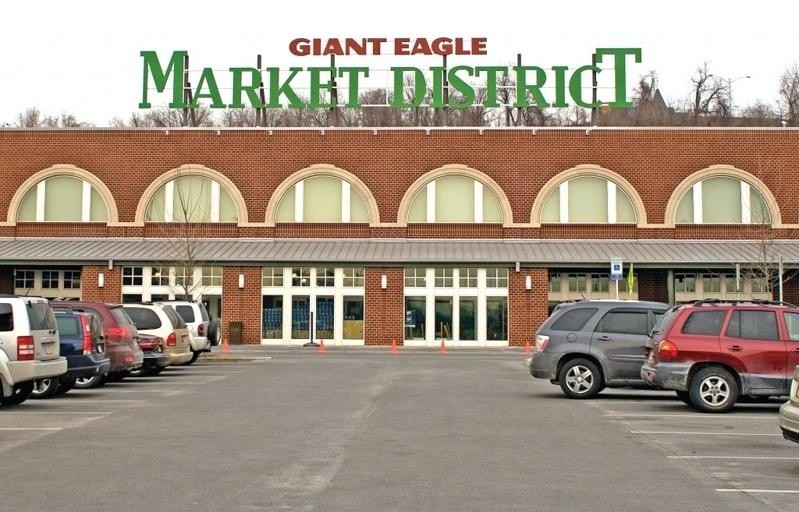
777, 361, 799, 444
139, 333, 171, 369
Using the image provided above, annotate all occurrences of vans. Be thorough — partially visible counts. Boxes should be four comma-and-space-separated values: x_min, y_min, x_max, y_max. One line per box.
114, 303, 195, 370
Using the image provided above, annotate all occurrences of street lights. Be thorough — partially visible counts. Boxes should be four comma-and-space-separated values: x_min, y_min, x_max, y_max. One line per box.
708, 72, 751, 115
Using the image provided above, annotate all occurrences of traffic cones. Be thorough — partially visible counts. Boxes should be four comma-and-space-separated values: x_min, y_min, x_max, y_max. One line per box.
220, 340, 229, 354
316, 340, 327, 352
389, 339, 399, 353
437, 339, 450, 354
524, 341, 532, 355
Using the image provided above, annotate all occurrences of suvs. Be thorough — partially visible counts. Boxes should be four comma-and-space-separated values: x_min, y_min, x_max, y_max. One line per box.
0, 283, 67, 409
29, 308, 110, 398
530, 299, 675, 399
45, 301, 144, 389
641, 298, 799, 413
143, 299, 224, 368
